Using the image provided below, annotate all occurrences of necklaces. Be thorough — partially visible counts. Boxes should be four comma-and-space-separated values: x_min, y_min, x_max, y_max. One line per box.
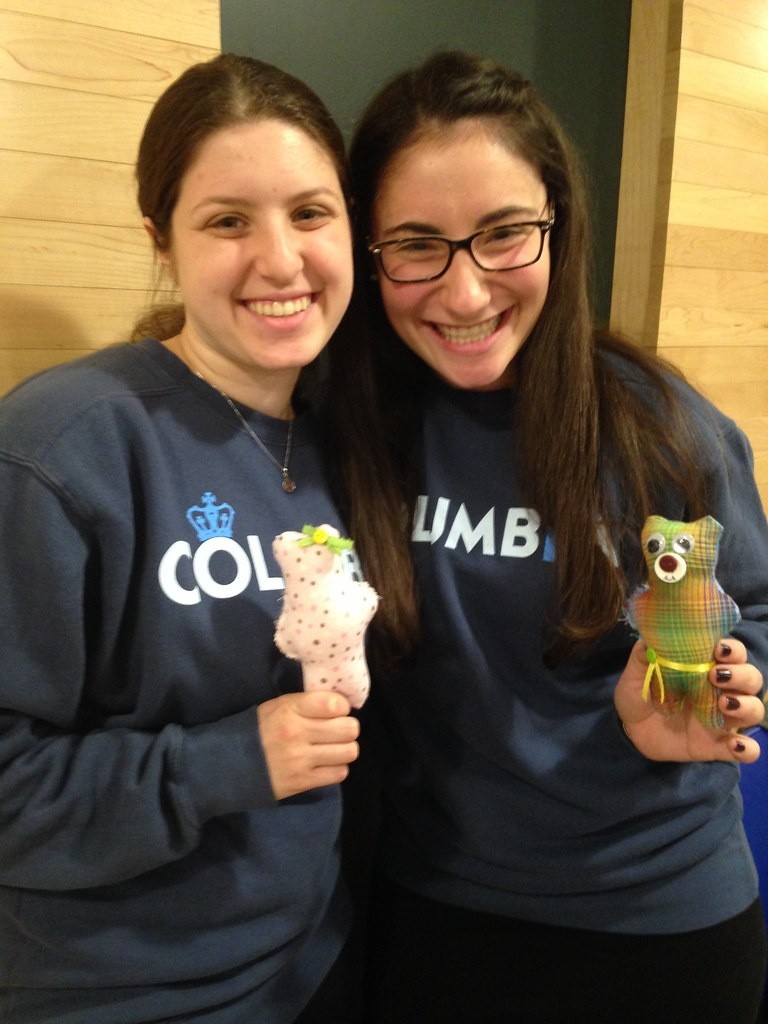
177, 333, 296, 493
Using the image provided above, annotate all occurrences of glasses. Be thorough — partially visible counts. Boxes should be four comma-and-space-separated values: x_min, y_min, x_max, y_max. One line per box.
366, 197, 556, 283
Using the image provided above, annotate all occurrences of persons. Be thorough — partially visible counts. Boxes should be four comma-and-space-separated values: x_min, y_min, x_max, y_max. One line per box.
308, 49, 768, 1024
0, 52, 379, 1024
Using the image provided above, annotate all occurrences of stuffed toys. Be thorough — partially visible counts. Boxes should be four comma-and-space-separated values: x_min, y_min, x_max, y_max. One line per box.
624, 514, 740, 731
273, 523, 379, 711
